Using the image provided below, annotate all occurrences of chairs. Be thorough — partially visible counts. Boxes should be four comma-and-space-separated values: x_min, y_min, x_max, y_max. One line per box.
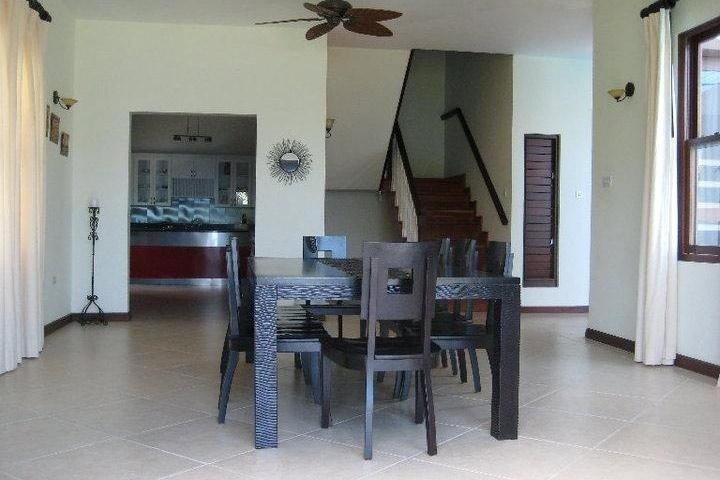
319, 241, 442, 461
301, 235, 346, 339
376, 237, 514, 402
217, 234, 331, 424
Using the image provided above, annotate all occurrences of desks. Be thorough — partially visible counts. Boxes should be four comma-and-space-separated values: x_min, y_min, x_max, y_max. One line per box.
246, 257, 520, 450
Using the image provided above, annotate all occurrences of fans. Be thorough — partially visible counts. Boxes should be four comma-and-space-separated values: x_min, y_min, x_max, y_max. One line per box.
254, 0, 406, 40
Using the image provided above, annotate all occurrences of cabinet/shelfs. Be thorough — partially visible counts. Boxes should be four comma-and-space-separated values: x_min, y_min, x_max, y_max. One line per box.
215, 155, 255, 208
134, 153, 173, 208
172, 154, 215, 199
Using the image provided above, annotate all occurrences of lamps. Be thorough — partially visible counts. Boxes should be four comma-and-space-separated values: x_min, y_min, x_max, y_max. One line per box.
604, 82, 636, 103
325, 118, 335, 138
170, 116, 213, 144
52, 90, 79, 111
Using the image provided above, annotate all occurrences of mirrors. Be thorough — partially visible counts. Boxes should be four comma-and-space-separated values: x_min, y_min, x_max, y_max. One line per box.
266, 138, 314, 187
